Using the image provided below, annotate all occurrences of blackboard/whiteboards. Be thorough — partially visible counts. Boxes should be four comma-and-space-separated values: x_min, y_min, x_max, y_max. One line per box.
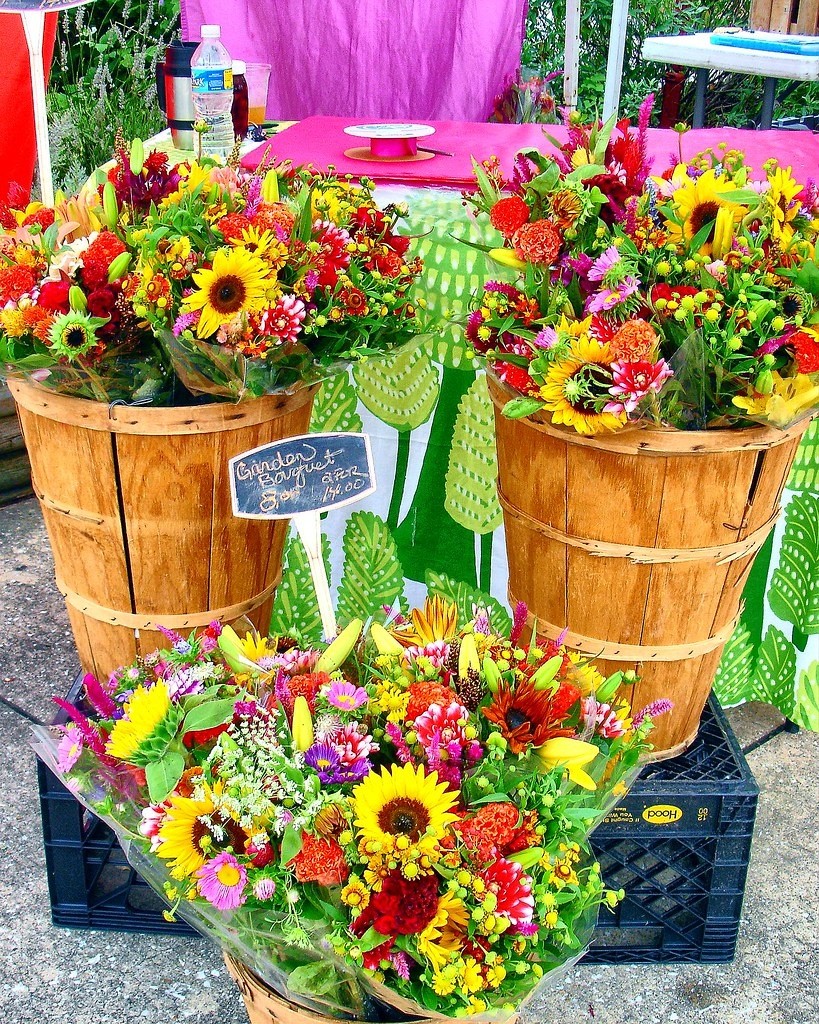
229, 432, 376, 520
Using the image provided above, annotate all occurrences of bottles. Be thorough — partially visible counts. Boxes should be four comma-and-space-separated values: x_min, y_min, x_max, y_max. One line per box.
190, 24, 237, 162
232, 59, 249, 143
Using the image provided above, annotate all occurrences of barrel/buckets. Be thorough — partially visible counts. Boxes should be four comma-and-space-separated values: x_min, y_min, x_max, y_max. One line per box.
485, 357, 818, 764
6, 364, 322, 700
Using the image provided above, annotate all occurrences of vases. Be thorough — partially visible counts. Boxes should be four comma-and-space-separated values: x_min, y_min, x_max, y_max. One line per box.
484, 372, 810, 770
5, 365, 327, 701
222, 953, 518, 1024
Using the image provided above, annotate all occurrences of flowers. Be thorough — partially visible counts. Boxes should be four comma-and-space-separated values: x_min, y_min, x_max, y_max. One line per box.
0, 116, 440, 408
444, 91, 819, 436
44, 592, 675, 1018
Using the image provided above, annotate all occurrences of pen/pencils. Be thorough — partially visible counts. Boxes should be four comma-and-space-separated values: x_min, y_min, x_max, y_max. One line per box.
266, 131, 281, 134
417, 147, 454, 158
250, 123, 279, 128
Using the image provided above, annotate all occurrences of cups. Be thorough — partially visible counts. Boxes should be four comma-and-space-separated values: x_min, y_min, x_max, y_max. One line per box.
243, 63, 271, 126
162, 41, 200, 151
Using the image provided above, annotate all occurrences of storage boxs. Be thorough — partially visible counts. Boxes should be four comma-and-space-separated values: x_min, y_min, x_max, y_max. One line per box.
35, 671, 760, 964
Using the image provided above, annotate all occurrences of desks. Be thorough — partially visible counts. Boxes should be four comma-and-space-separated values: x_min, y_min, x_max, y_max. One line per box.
640, 32, 819, 129
145, 118, 819, 748
